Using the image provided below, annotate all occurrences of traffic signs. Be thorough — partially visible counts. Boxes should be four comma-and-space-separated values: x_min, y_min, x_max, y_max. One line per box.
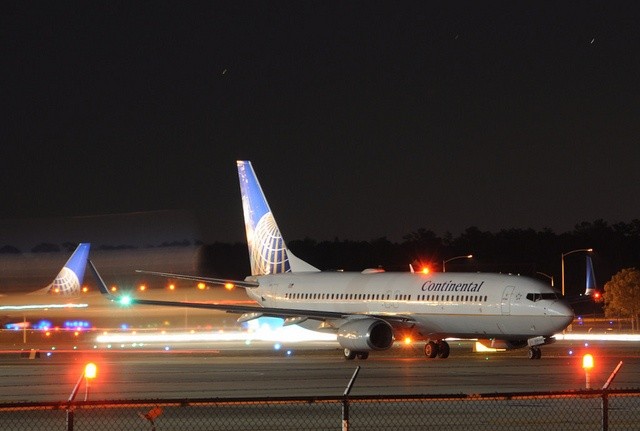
442, 255, 473, 273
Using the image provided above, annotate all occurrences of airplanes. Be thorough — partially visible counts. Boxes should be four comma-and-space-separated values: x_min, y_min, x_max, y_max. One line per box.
0, 243, 90, 299
87, 159, 574, 360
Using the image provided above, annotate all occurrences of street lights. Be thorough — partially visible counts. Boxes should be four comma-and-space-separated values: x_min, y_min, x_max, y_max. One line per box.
561, 248, 592, 297
535, 270, 554, 288
